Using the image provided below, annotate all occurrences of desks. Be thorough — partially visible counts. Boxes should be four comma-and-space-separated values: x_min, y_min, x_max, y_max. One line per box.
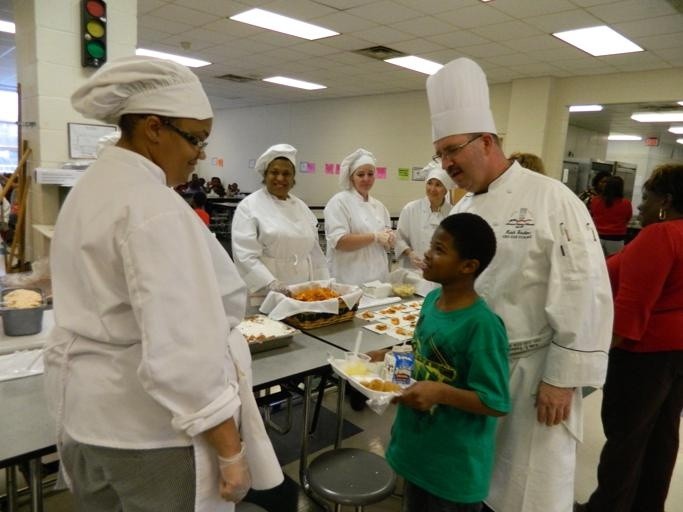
303, 296, 424, 436
0, 332, 345, 509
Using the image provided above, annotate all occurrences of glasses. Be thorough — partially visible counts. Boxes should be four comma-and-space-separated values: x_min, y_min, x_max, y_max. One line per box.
432, 134, 482, 162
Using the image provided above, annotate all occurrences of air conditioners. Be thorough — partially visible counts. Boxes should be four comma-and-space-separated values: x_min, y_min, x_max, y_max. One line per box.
353, 44, 408, 63
216, 73, 253, 85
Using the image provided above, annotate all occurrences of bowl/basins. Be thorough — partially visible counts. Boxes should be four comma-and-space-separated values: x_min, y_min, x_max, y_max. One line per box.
0, 285, 45, 338
391, 283, 416, 297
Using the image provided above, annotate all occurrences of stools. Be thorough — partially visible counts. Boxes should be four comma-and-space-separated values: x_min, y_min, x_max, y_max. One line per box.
307, 446, 396, 511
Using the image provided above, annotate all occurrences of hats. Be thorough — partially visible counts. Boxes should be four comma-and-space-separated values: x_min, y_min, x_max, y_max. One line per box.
338, 148, 376, 190
70, 56, 214, 123
426, 57, 498, 143
421, 157, 458, 193
255, 144, 297, 178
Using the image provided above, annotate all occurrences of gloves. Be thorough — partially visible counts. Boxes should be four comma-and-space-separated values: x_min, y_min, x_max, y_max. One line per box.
216, 443, 252, 503
409, 250, 428, 270
267, 281, 292, 298
374, 232, 396, 247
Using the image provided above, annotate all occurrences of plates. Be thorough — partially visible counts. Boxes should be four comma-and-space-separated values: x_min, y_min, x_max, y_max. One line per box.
244, 314, 301, 355
326, 358, 417, 400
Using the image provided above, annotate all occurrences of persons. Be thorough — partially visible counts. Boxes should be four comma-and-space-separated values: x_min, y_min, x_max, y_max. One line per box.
574, 163, 682, 511
588, 177, 633, 261
46, 57, 285, 512
363, 212, 512, 511
511, 153, 544, 174
176, 172, 241, 230
232, 144, 327, 298
396, 164, 463, 269
425, 55, 613, 512
575, 169, 610, 204
324, 150, 398, 274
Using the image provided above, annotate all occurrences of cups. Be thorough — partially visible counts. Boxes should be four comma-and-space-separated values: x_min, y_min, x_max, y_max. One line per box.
344, 351, 373, 376
